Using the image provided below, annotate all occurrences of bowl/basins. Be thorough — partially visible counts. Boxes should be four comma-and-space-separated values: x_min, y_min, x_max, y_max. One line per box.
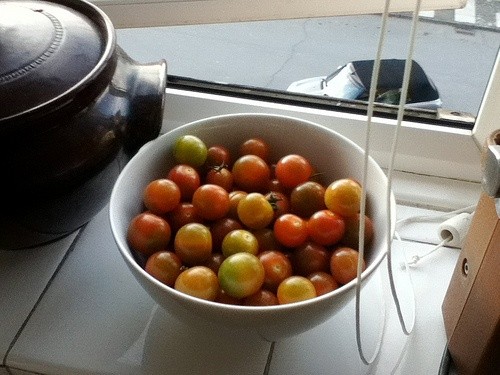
109, 113, 399, 342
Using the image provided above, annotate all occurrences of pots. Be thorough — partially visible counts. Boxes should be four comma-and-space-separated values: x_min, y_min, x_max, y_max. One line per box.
0, 0, 166, 249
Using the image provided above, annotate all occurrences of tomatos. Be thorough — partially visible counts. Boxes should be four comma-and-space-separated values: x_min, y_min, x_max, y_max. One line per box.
127, 134, 373, 305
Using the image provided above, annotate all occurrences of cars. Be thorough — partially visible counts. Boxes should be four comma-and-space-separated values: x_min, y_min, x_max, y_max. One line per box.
286, 57, 444, 117
